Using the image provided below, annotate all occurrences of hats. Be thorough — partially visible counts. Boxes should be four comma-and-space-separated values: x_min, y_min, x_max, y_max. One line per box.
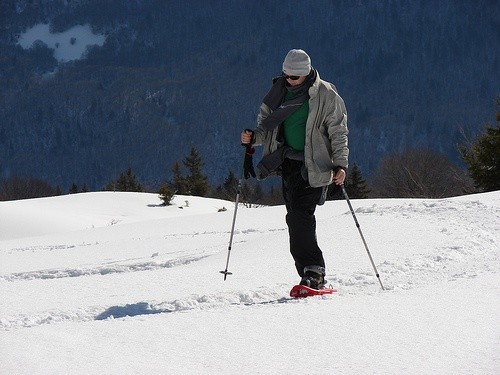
282, 49, 312, 76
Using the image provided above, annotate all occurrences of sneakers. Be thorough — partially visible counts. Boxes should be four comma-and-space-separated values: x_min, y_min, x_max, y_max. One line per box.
299, 270, 325, 289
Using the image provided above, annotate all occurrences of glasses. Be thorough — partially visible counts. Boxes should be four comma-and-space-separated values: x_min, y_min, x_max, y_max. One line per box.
282, 73, 301, 80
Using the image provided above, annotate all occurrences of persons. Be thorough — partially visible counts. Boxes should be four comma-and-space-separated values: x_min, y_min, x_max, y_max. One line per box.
240, 48, 349, 290
